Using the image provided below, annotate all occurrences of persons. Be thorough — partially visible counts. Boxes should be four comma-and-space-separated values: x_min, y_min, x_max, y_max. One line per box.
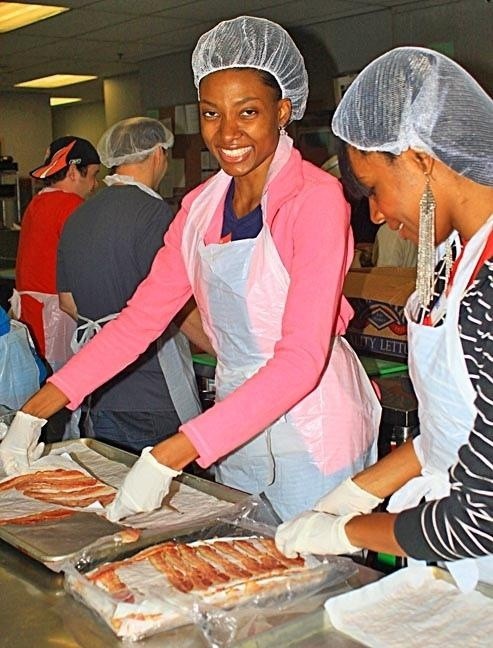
355, 221, 418, 270
275, 46, 493, 588
9, 136, 102, 444
57, 118, 218, 457
0, 305, 49, 444
1, 17, 383, 562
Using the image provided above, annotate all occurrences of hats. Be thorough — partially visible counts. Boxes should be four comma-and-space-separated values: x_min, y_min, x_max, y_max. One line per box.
28, 134, 102, 180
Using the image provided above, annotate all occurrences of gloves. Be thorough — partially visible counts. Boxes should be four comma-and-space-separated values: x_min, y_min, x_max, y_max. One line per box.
102, 444, 183, 523
273, 473, 385, 561
0, 407, 47, 478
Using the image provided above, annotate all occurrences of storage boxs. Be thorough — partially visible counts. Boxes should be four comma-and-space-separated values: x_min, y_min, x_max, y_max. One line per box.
341, 266, 418, 365
